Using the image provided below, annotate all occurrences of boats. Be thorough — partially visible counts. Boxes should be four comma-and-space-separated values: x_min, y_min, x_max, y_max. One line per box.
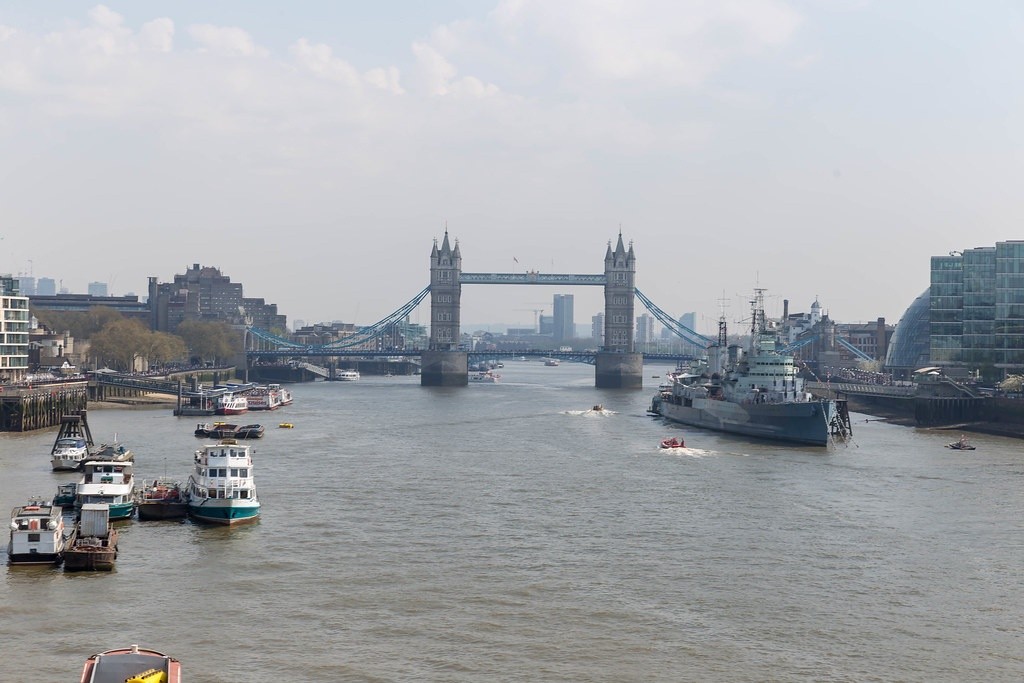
75, 457, 134, 519
137, 457, 188, 520
512, 356, 529, 362
468, 372, 501, 384
83, 433, 135, 466
80, 644, 181, 683
662, 439, 680, 449
337, 368, 359, 381
8, 496, 65, 563
52, 439, 87, 470
652, 287, 831, 447
217, 382, 293, 414
539, 356, 560, 366
210, 423, 241, 437
195, 423, 212, 434
949, 434, 975, 451
186, 440, 260, 524
594, 403, 603, 412
236, 424, 265, 438
53, 483, 76, 507
65, 504, 118, 571
